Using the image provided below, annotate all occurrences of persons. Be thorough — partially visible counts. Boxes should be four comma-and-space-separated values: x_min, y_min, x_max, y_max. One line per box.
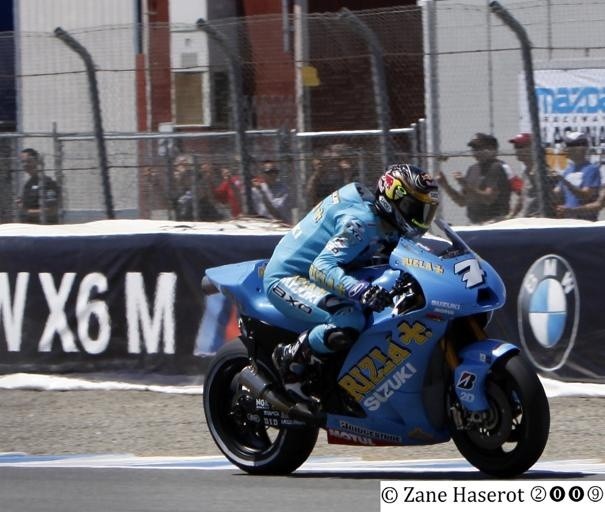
436, 131, 605, 225
262, 163, 441, 403
172, 144, 360, 227
15, 148, 64, 224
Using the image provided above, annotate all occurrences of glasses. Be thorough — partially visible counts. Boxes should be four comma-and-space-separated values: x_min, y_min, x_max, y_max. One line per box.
263, 167, 279, 174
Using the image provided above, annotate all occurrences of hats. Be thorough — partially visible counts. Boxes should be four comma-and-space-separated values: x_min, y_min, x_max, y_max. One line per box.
508, 133, 536, 144
563, 132, 589, 145
469, 133, 489, 147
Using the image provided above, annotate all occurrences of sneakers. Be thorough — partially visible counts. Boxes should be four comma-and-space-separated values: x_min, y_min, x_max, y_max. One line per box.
272, 343, 321, 415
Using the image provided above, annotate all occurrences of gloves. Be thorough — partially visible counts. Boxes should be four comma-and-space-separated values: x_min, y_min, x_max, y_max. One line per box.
347, 279, 393, 313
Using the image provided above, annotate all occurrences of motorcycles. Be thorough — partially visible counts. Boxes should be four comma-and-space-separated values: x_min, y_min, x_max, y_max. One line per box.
195, 220, 559, 479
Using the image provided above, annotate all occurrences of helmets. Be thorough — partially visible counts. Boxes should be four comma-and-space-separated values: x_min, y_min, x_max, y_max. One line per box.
372, 163, 441, 238
